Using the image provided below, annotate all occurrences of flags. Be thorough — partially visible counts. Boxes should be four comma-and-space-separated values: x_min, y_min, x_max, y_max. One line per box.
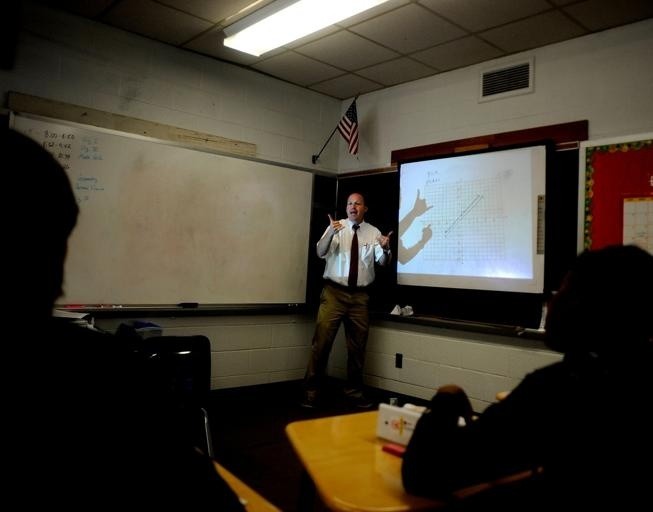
334, 99, 359, 159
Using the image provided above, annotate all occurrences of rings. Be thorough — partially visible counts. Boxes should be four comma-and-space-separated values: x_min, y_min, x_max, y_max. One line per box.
380, 238, 384, 242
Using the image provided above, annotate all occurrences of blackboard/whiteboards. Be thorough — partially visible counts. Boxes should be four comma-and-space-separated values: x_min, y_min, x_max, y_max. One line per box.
10, 110, 313, 314
337, 141, 579, 331
305, 170, 337, 304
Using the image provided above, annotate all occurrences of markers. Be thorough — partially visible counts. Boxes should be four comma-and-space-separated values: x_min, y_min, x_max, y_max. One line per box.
176, 303, 198, 308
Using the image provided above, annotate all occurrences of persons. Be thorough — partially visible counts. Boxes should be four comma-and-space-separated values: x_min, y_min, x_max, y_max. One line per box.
398, 189, 434, 265
0, 123, 248, 511
398, 243, 652, 511
296, 191, 375, 409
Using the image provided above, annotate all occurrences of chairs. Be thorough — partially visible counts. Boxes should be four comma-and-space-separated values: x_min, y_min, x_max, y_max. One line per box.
141, 334, 214, 459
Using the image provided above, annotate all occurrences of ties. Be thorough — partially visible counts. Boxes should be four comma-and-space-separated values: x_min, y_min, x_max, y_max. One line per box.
348, 224, 360, 287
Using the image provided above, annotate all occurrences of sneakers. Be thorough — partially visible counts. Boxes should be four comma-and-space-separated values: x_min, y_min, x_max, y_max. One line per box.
345, 389, 371, 407
303, 391, 315, 408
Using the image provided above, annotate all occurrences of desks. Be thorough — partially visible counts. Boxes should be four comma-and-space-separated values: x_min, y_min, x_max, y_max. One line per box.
285, 406, 541, 511
